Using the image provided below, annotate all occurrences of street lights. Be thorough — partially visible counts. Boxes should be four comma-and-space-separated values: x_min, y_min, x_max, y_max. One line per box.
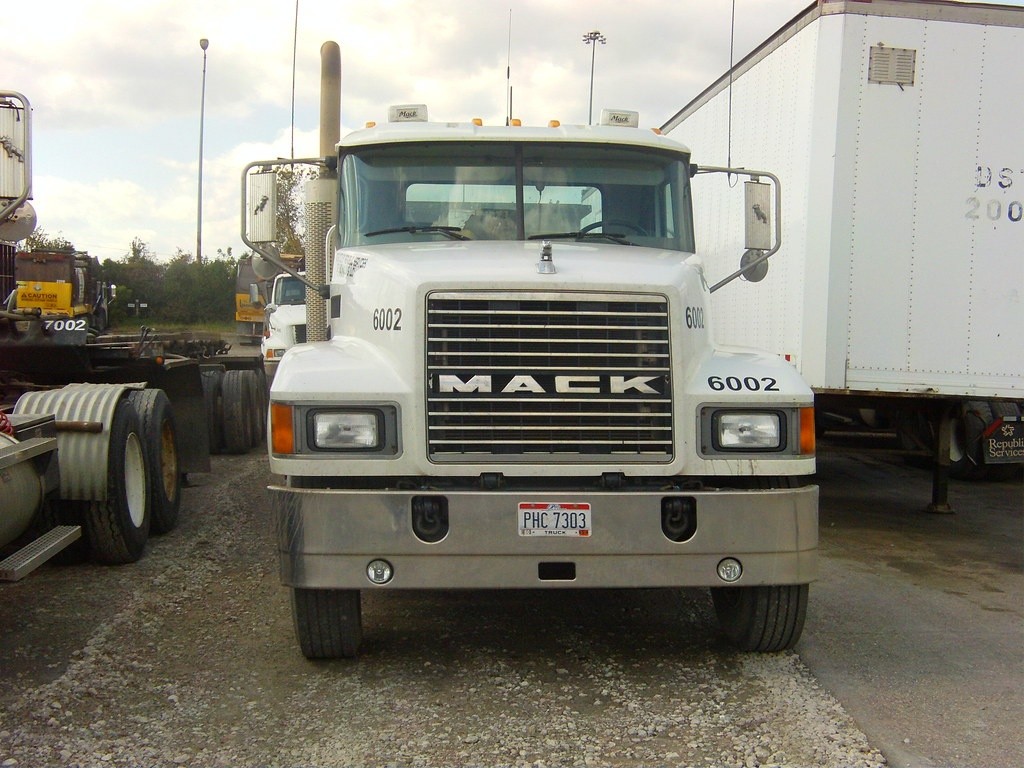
194, 38, 210, 267
583, 31, 606, 125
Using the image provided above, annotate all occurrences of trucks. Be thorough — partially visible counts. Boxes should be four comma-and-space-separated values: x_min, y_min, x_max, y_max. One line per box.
249, 272, 331, 377
265, 96, 818, 661
657, 0, 1023, 517
8, 248, 113, 341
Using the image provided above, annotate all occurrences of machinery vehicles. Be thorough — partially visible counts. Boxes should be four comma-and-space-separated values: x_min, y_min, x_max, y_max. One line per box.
233, 249, 308, 343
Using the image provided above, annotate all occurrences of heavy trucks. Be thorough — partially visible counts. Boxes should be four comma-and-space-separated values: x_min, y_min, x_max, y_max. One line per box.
1, 88, 270, 588
